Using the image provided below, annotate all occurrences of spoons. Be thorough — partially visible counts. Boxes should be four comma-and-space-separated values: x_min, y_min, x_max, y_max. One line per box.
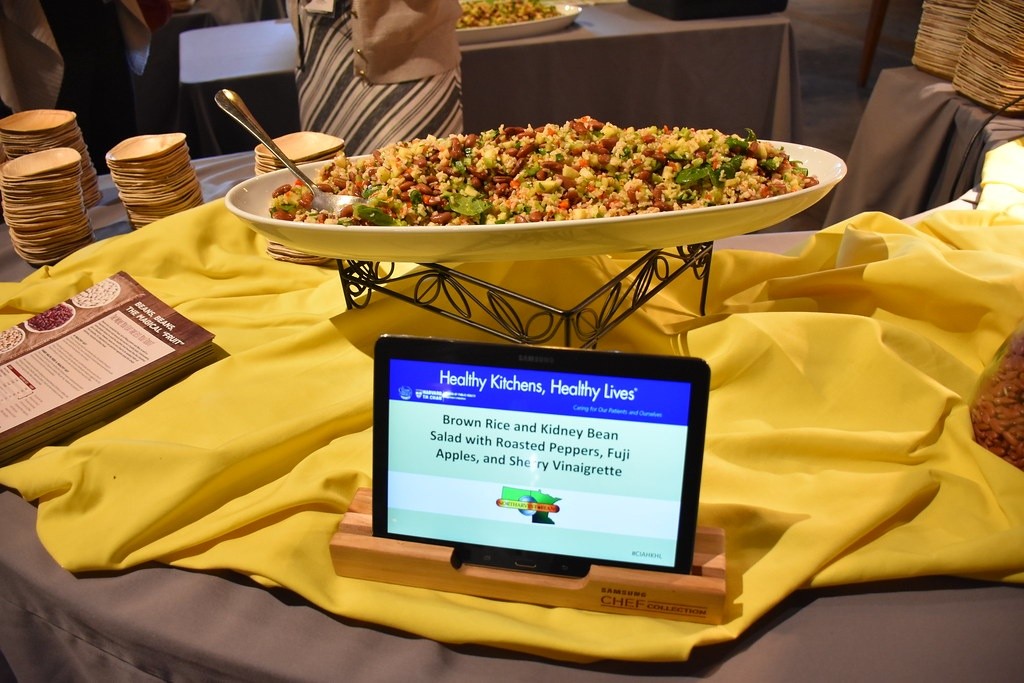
214, 89, 369, 215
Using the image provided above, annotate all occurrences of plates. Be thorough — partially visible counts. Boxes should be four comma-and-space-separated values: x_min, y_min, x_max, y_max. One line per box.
224, 137, 847, 264
455, 2, 582, 44
0, 107, 347, 268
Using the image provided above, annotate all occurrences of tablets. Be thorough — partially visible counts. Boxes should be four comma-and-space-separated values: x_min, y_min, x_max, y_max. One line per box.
372, 333, 711, 578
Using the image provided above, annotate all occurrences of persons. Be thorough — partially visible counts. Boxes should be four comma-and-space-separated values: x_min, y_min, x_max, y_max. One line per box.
284, 0, 465, 157
0, 0, 153, 176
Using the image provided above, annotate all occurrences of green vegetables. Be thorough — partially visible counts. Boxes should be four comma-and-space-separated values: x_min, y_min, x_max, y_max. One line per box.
270, 127, 811, 228
